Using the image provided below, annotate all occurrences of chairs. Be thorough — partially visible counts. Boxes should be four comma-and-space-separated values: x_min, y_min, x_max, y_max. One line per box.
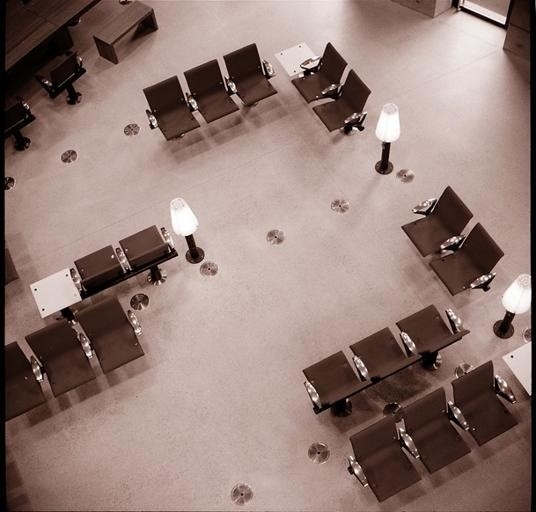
36, 49, 86, 105
5, 341, 47, 421
451, 360, 519, 446
429, 222, 505, 297
312, 69, 372, 134
183, 59, 240, 123
291, 42, 348, 104
402, 386, 472, 474
224, 43, 278, 106
73, 245, 124, 296
302, 350, 373, 417
75, 296, 145, 374
25, 321, 96, 398
349, 327, 422, 394
119, 225, 179, 286
142, 75, 201, 141
348, 411, 423, 503
401, 185, 473, 257
396, 304, 471, 370
92, 0, 158, 64
3, 96, 36, 151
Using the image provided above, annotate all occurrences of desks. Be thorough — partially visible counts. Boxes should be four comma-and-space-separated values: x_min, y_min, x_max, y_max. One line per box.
5, 0, 97, 70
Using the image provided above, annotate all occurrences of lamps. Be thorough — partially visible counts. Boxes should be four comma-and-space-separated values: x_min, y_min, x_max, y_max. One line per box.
375, 103, 401, 175
492, 274, 532, 339
170, 197, 206, 264
